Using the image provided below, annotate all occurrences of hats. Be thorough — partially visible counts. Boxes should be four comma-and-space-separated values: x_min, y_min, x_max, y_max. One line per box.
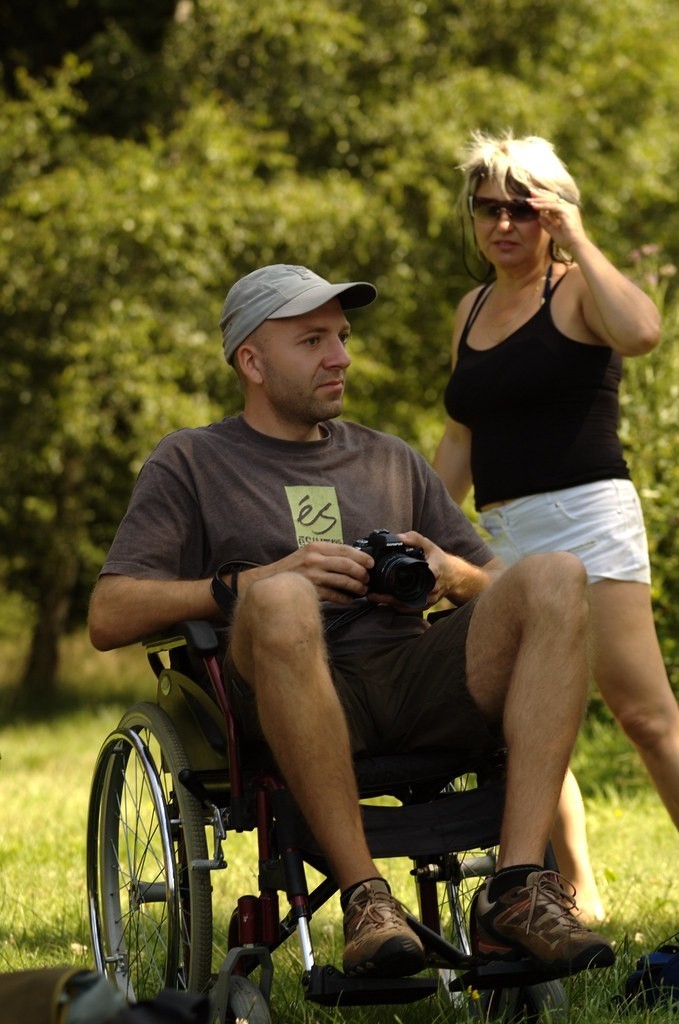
220, 264, 378, 365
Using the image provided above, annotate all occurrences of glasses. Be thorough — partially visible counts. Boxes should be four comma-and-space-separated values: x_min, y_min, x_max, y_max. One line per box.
468, 195, 541, 223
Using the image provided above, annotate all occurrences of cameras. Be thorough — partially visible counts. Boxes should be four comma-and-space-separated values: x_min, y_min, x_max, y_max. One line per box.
352, 529, 435, 609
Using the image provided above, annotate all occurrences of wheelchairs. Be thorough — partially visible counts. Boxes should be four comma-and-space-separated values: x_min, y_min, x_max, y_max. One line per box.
83, 625, 569, 1023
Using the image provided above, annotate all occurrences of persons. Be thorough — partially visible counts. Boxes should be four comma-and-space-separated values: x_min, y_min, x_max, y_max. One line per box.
89, 262, 616, 973
433, 135, 679, 928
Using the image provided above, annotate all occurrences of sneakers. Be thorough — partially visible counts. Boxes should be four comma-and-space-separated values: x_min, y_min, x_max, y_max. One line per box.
342, 878, 431, 979
470, 870, 617, 970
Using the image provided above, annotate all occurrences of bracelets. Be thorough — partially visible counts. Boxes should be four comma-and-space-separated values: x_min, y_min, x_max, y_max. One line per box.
230, 568, 239, 596
